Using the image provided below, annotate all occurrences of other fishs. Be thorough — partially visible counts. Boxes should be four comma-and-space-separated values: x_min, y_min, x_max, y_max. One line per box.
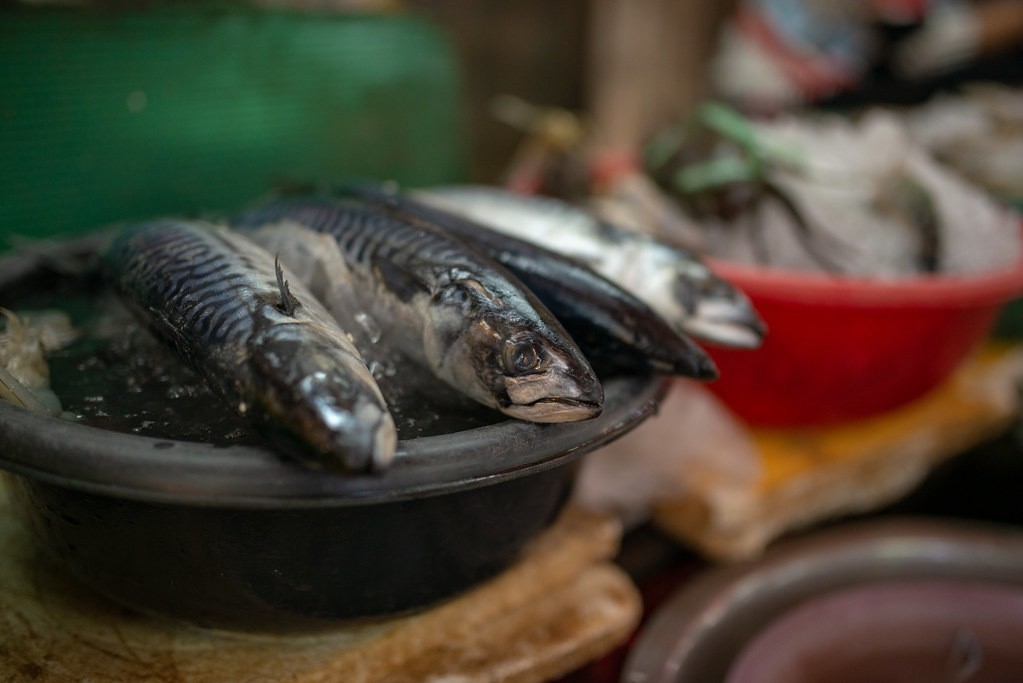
113, 186, 769, 476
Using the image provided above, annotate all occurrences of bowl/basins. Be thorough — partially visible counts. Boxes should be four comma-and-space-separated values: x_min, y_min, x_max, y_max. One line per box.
0, 305, 676, 615
664, 222, 1021, 426
619, 526, 1022, 679
